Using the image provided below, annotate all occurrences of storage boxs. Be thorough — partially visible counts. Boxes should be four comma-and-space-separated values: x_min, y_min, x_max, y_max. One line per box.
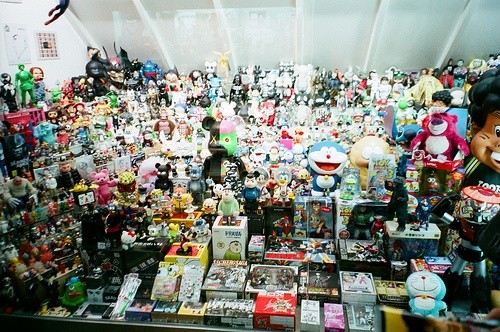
73, 155, 131, 177
169, 177, 191, 189
73, 190, 500, 332
34, 165, 60, 184
445, 107, 468, 140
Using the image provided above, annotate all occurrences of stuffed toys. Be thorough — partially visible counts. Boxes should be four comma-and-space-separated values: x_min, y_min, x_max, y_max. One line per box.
0, 46, 500, 332
